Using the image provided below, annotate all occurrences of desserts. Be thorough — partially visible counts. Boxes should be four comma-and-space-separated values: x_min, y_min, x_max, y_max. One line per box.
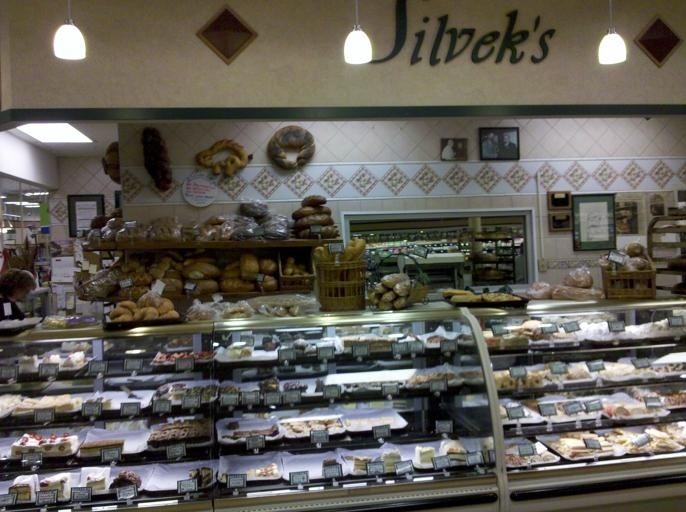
0, 309, 686, 504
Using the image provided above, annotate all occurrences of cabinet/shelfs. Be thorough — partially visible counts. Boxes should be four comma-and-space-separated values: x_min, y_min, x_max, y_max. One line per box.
89, 239, 344, 299
645, 216, 686, 291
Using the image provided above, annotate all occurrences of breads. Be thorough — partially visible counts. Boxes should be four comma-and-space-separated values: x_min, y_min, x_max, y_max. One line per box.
76, 194, 366, 320
442, 288, 521, 303
527, 242, 652, 302
369, 274, 411, 310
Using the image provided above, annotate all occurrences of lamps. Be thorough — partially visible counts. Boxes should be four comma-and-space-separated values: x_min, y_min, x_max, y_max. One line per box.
598, 1, 628, 66
54, 0, 87, 62
343, 1, 373, 65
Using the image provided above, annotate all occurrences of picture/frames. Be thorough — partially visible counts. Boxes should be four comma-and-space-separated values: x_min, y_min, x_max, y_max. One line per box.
478, 126, 521, 161
572, 192, 617, 252
67, 194, 105, 237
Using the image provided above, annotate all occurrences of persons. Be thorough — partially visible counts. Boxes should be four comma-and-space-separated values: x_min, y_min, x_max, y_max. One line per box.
441, 139, 457, 160
0, 268, 37, 320
481, 127, 518, 158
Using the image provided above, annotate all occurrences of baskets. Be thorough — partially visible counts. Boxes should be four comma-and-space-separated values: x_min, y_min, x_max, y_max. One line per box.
601, 247, 658, 298
278, 247, 429, 310
